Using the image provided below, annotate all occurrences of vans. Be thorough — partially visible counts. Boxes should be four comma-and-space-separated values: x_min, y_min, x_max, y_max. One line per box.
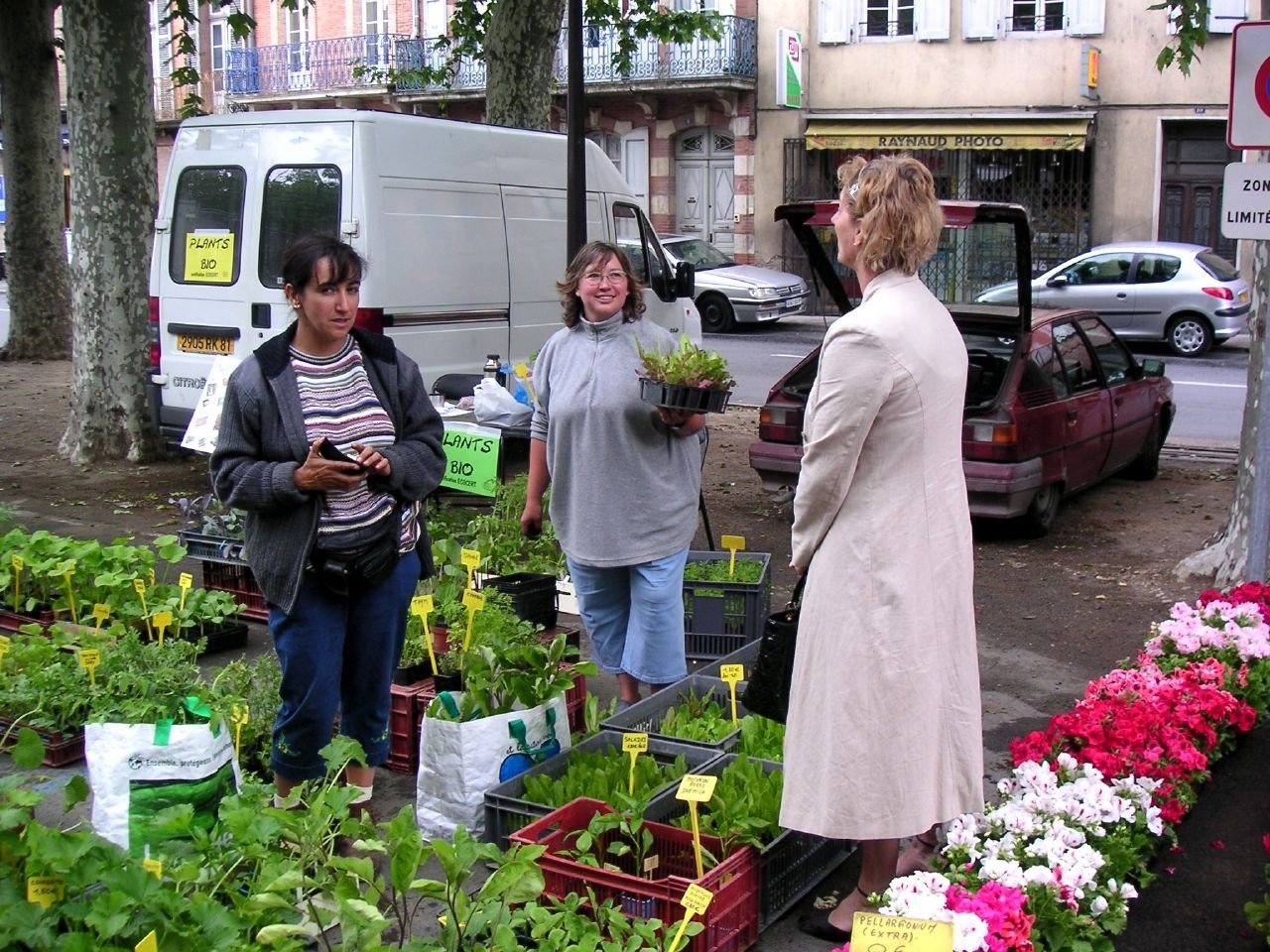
146, 109, 702, 446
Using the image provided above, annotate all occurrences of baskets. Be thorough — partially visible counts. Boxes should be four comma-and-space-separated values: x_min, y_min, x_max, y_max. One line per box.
482, 572, 571, 632
0, 524, 250, 768
638, 377, 733, 414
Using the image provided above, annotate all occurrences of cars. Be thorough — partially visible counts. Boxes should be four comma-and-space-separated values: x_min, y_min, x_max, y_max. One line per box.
614, 232, 811, 334
972, 241, 1251, 359
748, 199, 1176, 541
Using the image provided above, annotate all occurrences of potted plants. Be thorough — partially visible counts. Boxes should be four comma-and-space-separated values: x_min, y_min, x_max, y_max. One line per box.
635, 333, 736, 414
0, 472, 857, 952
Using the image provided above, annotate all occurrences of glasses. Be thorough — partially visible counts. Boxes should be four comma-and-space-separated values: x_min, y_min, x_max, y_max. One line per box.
583, 271, 627, 285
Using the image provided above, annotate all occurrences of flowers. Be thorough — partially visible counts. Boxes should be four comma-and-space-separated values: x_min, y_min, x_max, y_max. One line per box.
831, 581, 1270, 952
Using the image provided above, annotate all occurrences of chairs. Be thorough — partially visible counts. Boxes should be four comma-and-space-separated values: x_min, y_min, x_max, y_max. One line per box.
1117, 259, 1151, 283
427, 373, 506, 516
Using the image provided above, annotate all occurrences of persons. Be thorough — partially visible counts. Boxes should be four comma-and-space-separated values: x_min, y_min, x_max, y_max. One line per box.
777, 156, 986, 941
522, 241, 707, 712
208, 234, 448, 883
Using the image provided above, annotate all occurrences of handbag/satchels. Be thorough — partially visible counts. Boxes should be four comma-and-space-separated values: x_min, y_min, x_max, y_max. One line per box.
473, 373, 533, 427
416, 691, 572, 846
314, 505, 402, 599
83, 696, 245, 851
740, 569, 809, 725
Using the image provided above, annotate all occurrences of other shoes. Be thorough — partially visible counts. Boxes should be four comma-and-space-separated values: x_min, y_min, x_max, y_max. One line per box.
798, 885, 883, 943
269, 799, 306, 811
338, 800, 380, 879
617, 694, 641, 714
